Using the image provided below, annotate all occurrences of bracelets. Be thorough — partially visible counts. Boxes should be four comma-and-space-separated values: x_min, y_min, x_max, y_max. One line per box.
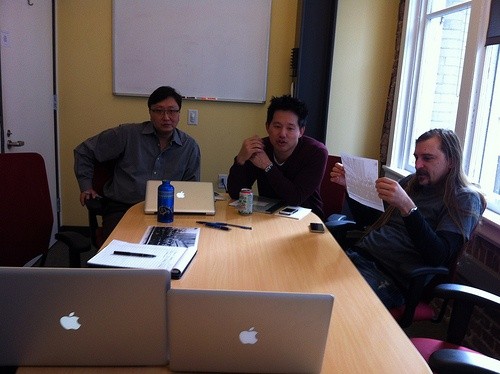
408, 207, 417, 215
265, 164, 274, 172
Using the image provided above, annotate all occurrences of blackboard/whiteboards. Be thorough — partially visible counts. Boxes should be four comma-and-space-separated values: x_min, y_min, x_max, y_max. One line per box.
111, 0, 272, 104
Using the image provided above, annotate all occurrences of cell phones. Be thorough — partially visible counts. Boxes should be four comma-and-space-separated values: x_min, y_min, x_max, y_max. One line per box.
310, 223, 324, 232
279, 207, 298, 214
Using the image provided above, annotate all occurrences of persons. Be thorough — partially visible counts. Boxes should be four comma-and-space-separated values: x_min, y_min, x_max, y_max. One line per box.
226, 93, 330, 219
329, 128, 483, 292
73, 86, 200, 242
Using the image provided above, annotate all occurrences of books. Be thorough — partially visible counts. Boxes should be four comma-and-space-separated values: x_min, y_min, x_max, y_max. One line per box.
86, 239, 198, 280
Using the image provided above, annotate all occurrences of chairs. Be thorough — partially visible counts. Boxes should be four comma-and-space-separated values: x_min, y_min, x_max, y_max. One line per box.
321, 155, 345, 214
0, 152, 110, 267
390, 266, 500, 374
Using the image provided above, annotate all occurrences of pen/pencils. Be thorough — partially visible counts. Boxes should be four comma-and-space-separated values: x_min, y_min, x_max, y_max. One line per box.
196, 221, 252, 230
114, 251, 156, 258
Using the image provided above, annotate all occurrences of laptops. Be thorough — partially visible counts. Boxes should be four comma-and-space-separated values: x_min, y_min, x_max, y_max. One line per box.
145, 180, 216, 215
165, 288, 333, 374
0, 267, 171, 366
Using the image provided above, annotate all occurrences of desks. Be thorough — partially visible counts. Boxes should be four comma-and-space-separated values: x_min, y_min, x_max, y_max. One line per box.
15, 193, 433, 374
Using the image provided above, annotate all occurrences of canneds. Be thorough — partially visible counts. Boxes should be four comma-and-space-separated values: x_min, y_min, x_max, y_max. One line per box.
238, 188, 253, 215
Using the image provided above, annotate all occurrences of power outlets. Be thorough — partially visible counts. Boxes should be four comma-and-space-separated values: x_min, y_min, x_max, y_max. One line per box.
188, 109, 198, 125
219, 175, 227, 188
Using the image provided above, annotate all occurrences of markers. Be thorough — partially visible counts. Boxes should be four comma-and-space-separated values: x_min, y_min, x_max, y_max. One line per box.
202, 97, 217, 100
185, 96, 196, 99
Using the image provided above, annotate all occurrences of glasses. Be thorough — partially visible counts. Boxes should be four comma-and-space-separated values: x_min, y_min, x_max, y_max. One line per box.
149, 108, 180, 116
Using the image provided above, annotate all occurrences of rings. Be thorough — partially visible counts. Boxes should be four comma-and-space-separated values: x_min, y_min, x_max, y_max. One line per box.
86, 192, 89, 195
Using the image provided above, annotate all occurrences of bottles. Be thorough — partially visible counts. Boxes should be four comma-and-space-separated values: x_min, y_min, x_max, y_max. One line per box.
157, 180, 174, 222
